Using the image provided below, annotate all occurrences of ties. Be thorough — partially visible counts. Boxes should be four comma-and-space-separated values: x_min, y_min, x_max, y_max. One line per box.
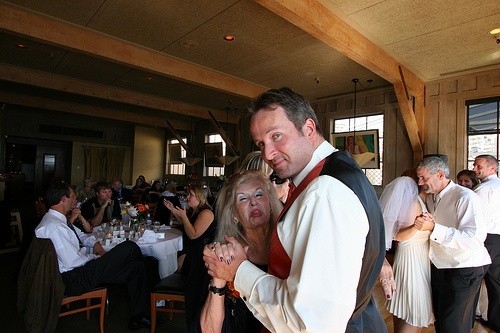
65, 216, 84, 248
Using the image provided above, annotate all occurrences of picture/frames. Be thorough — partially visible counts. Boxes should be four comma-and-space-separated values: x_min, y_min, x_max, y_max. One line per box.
331, 129, 381, 169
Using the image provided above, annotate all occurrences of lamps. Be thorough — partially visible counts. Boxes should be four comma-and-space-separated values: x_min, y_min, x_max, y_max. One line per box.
214, 107, 240, 165
351, 78, 378, 168
177, 118, 202, 167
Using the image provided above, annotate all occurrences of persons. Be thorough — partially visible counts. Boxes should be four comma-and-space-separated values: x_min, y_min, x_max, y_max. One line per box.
380, 167, 436, 332
66, 177, 133, 232
457, 170, 483, 318
471, 155, 500, 333
200, 169, 285, 332
132, 175, 182, 226
203, 86, 397, 333
237, 150, 291, 207
34, 186, 159, 330
163, 185, 216, 332
414, 154, 492, 333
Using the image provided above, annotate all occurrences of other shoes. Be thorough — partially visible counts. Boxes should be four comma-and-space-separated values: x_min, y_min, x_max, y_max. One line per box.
128, 314, 160, 331
480, 316, 500, 333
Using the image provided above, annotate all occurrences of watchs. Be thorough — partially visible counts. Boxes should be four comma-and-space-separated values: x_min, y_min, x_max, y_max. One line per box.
208, 279, 229, 296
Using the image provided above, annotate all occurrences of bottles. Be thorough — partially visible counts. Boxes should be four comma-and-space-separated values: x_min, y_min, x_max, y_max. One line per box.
146, 215, 152, 225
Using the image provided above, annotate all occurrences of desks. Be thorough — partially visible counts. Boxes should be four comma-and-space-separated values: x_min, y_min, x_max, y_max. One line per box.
85, 223, 184, 316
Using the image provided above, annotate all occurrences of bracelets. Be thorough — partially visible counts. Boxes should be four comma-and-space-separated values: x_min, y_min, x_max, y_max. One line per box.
82, 221, 88, 226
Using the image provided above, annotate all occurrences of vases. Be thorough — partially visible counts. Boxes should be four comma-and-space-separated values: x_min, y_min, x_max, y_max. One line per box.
128, 218, 139, 232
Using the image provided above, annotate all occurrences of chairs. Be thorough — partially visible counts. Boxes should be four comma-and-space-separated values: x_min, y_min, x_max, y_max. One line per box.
21, 193, 189, 333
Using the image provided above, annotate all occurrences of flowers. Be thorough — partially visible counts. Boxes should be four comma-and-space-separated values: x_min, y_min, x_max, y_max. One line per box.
119, 200, 150, 222
226, 281, 240, 304
